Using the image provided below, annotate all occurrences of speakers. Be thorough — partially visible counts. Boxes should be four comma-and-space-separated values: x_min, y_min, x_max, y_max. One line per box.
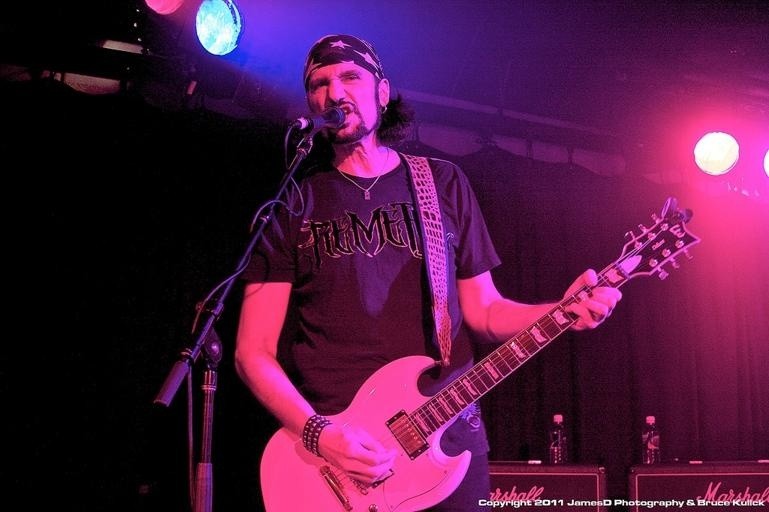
487, 464, 607, 512
628, 463, 769, 512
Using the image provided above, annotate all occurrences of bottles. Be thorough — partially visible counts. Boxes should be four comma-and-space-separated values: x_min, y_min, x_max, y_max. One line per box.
547, 413, 569, 465
641, 414, 662, 466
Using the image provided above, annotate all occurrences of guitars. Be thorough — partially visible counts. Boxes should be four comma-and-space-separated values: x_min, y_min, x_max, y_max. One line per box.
258, 199, 700, 512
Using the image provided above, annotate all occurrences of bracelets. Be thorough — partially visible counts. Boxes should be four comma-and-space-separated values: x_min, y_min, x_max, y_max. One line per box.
302, 414, 333, 457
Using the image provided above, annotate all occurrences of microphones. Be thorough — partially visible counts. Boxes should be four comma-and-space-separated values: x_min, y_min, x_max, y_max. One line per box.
288, 106, 346, 128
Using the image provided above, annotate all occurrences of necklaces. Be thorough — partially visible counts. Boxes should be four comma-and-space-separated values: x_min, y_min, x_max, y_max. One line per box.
333, 146, 390, 201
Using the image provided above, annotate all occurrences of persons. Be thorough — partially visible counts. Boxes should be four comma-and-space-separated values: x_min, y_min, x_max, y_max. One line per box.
234, 34, 623, 512
